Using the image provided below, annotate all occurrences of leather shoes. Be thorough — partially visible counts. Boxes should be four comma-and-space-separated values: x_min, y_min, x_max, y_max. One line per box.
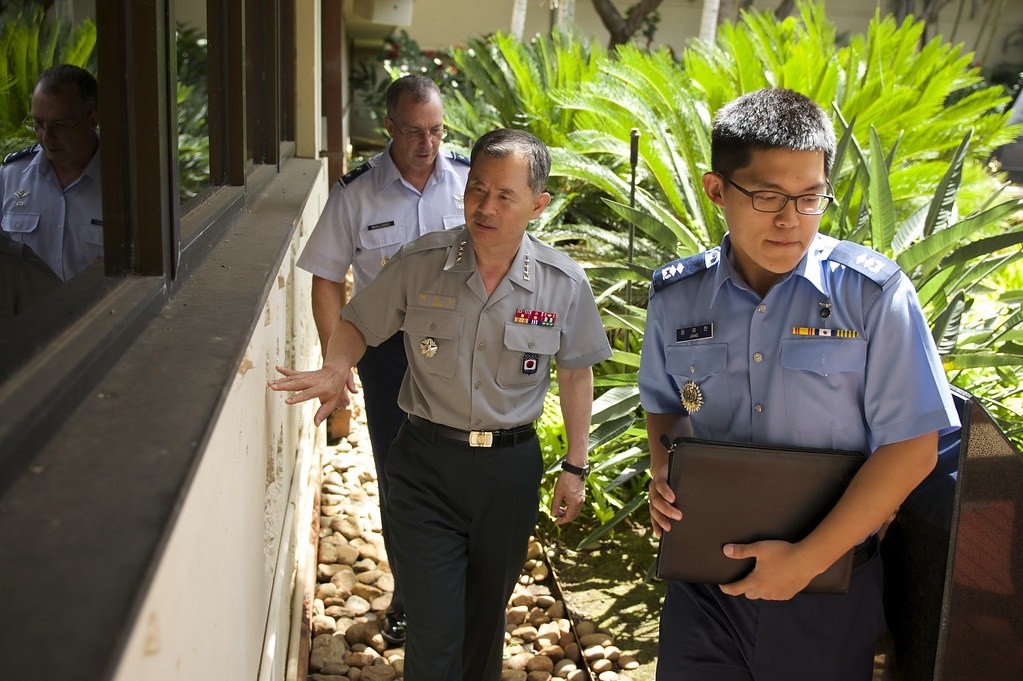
380, 602, 407, 643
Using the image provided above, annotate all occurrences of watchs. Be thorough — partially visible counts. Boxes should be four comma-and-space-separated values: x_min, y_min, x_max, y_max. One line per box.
561, 461, 590, 481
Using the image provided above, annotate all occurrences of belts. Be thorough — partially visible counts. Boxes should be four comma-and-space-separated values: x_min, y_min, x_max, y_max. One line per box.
852, 533, 877, 570
409, 414, 536, 449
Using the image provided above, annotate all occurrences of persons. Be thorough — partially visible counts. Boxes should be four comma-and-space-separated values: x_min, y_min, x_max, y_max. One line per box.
637, 90, 962, 681
296, 73, 479, 646
266, 129, 611, 681
0, 62, 107, 283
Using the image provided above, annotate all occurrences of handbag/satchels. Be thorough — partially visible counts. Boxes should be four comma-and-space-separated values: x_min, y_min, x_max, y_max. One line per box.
655, 437, 865, 597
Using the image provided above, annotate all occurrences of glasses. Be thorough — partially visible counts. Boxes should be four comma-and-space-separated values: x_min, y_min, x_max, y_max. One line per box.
22, 110, 92, 135
723, 175, 835, 215
391, 118, 448, 141
